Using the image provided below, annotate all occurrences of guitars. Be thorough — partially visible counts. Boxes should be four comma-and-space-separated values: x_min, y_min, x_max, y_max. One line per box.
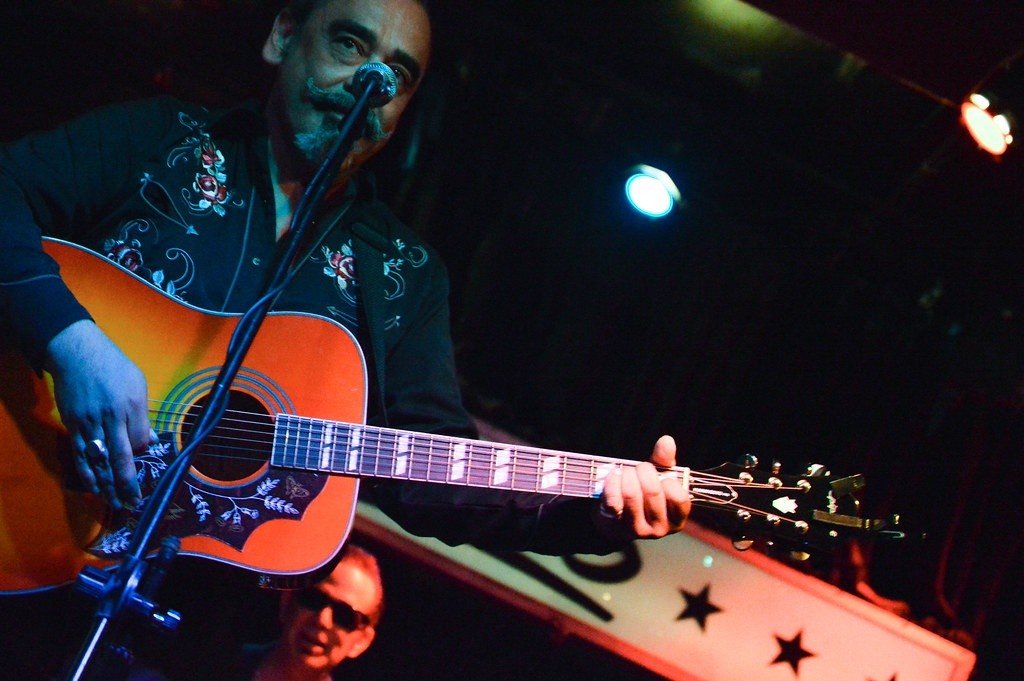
1, 228, 910, 610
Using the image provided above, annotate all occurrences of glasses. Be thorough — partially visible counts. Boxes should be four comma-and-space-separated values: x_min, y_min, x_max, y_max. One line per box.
294, 585, 370, 631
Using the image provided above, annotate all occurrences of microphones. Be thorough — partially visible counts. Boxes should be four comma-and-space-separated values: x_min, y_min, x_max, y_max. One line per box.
353, 61, 397, 108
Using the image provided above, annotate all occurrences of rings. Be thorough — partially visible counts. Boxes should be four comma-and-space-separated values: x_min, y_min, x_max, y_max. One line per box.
85, 439, 107, 456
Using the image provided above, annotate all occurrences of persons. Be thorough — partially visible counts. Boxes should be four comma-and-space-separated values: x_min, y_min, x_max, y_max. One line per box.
0, 0, 693, 541
245, 548, 386, 680
